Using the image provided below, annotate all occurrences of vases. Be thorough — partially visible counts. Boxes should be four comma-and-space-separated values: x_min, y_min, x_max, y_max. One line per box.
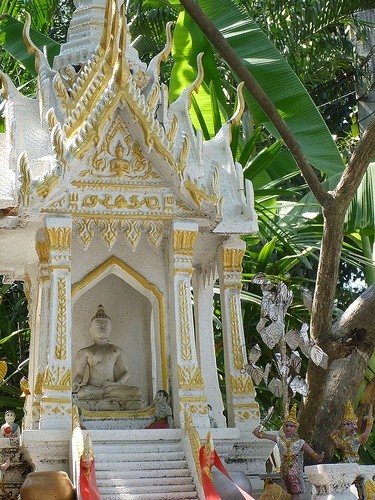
210, 470, 253, 500
20, 470, 77, 500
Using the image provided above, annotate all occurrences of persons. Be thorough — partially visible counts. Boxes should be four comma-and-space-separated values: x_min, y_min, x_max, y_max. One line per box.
144, 389, 175, 429
0, 409, 21, 439
71, 304, 149, 411
329, 399, 374, 465
252, 403, 325, 500
